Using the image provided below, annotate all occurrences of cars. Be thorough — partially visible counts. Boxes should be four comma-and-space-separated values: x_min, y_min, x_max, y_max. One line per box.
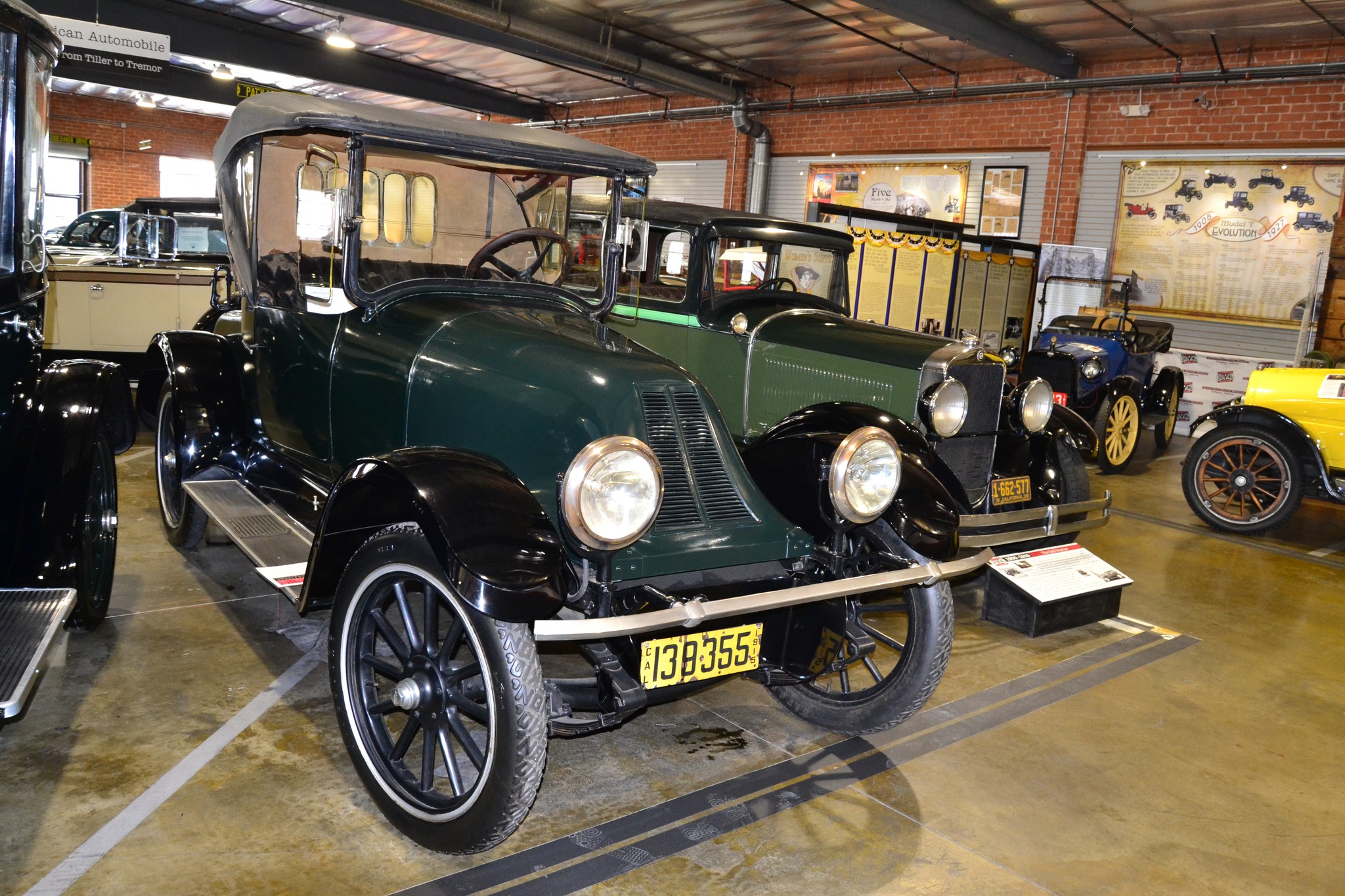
0, 0, 1191, 860
996, 274, 1184, 475
139, 91, 999, 859
1179, 363, 1345, 538
520, 189, 1114, 594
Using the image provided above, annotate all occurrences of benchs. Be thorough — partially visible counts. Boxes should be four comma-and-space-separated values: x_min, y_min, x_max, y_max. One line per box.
1050, 327, 1158, 354
617, 287, 710, 300
256, 251, 512, 312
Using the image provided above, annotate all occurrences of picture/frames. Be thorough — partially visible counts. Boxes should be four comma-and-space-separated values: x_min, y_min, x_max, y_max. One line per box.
977, 165, 1028, 240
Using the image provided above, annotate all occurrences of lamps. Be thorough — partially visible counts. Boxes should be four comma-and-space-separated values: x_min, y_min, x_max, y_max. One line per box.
211, 63, 234, 79
327, 16, 355, 48
136, 93, 157, 107
1119, 104, 1150, 116
